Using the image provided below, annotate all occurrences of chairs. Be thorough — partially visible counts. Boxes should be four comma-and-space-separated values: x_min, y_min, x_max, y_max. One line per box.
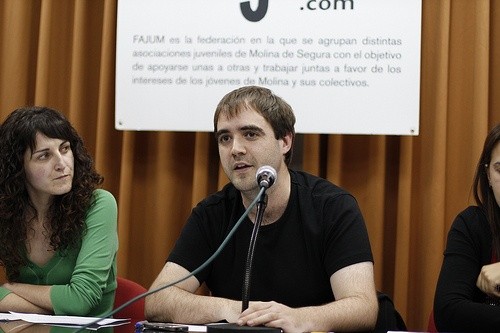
112, 276, 148, 333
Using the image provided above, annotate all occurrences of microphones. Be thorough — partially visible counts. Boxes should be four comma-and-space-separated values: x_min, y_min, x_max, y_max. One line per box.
255, 166, 277, 189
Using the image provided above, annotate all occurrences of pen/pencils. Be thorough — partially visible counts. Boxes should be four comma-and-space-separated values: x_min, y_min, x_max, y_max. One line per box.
135, 320, 148, 330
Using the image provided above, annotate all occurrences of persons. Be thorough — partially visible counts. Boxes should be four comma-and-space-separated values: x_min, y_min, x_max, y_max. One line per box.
143, 85, 407, 332
432, 125, 500, 333
0, 106, 119, 333
